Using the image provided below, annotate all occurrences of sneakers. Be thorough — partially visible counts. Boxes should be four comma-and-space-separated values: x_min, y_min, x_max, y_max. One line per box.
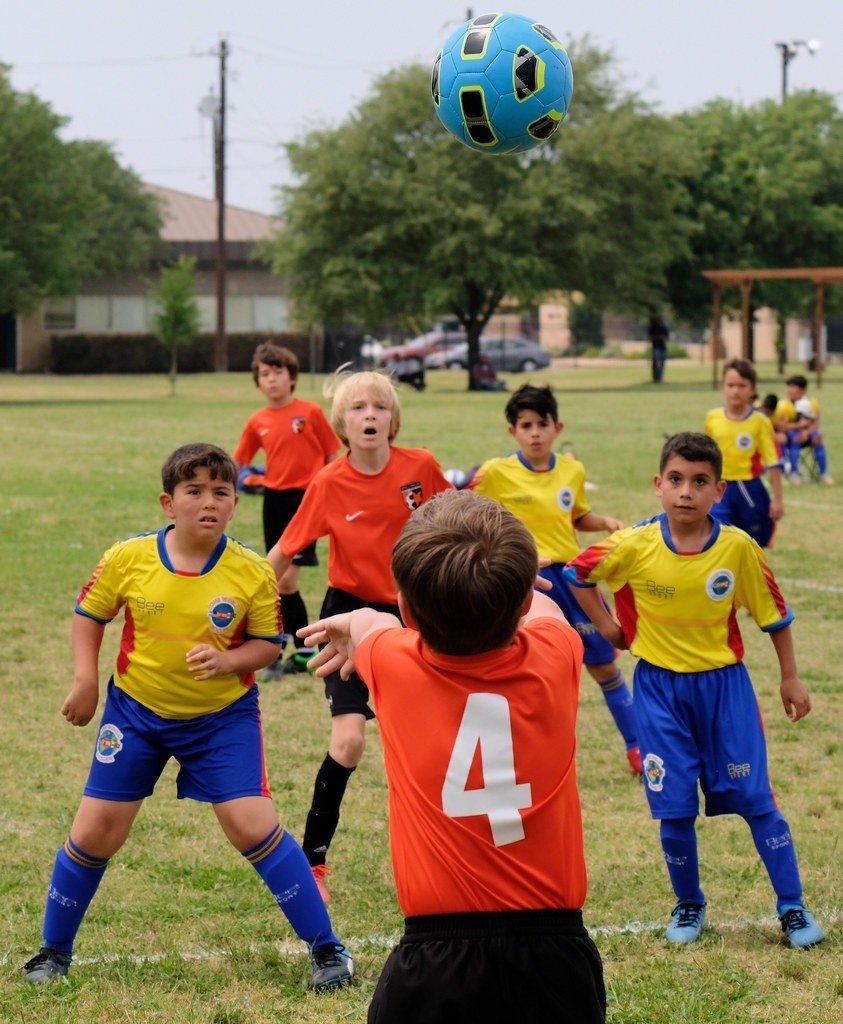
311, 864, 331, 905
24, 947, 72, 985
313, 943, 354, 988
778, 904, 824, 948
666, 899, 707, 944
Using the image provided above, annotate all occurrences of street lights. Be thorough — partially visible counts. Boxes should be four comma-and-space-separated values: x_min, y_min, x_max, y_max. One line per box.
776, 38, 822, 103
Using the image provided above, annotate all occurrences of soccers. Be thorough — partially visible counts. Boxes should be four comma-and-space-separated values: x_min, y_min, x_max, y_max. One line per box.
427, 11, 575, 154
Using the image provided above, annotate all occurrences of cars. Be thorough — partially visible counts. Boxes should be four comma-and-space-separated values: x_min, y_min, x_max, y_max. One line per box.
424, 336, 551, 374
381, 332, 470, 373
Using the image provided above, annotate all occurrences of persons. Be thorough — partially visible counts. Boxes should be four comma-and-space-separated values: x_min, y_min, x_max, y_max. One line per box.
228, 342, 343, 683
264, 372, 457, 903
473, 353, 507, 392
705, 359, 785, 617
562, 432, 826, 948
752, 375, 835, 486
18, 443, 355, 990
457, 387, 643, 776
647, 315, 669, 384
387, 351, 426, 391
296, 490, 607, 1024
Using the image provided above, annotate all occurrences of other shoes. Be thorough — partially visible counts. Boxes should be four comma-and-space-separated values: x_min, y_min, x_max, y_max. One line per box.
627, 747, 643, 773
261, 653, 317, 682
